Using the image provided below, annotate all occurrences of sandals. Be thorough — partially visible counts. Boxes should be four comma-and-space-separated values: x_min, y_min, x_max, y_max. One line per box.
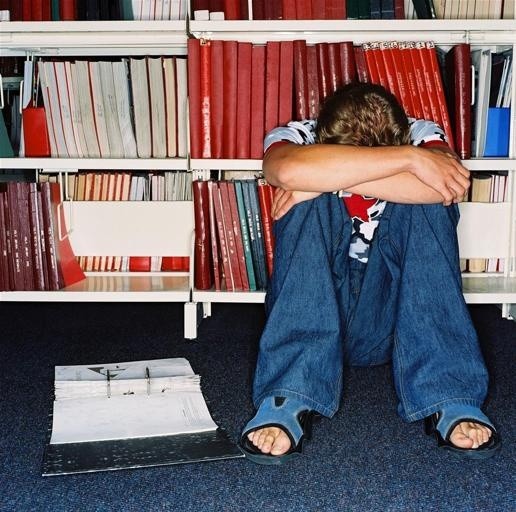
237, 396, 324, 465
421, 405, 502, 460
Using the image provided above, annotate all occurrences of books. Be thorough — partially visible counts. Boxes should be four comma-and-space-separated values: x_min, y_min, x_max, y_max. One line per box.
0, 168, 192, 293
0, 56, 192, 156
457, 175, 516, 274
200, 170, 267, 292
3, 0, 511, 25
192, 38, 507, 155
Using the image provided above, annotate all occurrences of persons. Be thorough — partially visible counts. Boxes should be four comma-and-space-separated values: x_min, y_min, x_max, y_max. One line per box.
236, 82, 500, 461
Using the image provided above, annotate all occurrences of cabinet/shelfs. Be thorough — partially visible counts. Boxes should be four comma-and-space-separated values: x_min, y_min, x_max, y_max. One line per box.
0, 21, 189, 339
189, 20, 513, 339
